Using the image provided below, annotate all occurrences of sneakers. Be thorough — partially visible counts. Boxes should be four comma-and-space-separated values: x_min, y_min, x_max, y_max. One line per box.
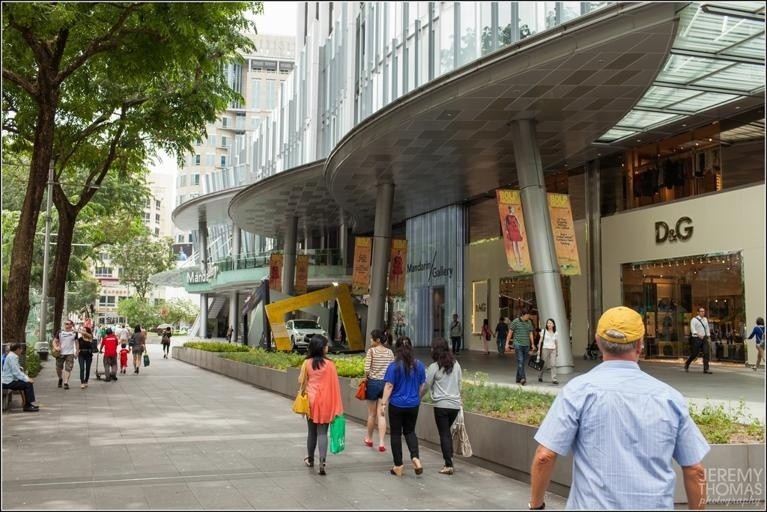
106, 377, 110, 382
110, 374, 117, 380
57, 379, 63, 388
80, 383, 84, 389
63, 383, 70, 389
515, 380, 520, 383
84, 383, 87, 387
539, 378, 543, 383
519, 378, 527, 386
553, 381, 559, 384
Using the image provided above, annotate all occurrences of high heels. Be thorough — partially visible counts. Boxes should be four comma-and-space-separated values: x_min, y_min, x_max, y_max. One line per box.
437, 466, 453, 475
389, 465, 403, 476
412, 456, 423, 476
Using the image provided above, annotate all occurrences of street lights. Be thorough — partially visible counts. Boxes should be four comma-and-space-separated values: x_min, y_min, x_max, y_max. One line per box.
30, 161, 107, 360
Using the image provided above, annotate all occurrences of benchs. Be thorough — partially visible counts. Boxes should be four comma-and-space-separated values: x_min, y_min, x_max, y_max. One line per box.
37, 349, 49, 360
2, 389, 25, 410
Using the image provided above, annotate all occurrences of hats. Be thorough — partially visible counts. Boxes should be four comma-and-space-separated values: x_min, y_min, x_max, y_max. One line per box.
594, 305, 645, 344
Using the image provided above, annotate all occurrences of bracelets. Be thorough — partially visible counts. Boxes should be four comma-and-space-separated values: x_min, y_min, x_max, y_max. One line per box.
529, 503, 545, 511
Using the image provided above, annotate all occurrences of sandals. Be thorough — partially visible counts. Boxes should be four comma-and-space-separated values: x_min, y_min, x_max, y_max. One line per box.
378, 446, 386, 452
303, 456, 313, 467
318, 463, 326, 475
363, 436, 373, 447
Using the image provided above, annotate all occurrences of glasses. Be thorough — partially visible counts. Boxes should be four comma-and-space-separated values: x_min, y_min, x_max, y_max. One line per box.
698, 310, 705, 313
65, 322, 69, 325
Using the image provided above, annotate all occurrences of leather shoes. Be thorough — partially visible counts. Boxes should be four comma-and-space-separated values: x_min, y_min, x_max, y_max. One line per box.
22, 405, 39, 412
30, 404, 39, 408
704, 369, 713, 374
684, 362, 689, 370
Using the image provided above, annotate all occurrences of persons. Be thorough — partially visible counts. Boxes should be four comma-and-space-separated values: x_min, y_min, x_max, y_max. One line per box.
481, 319, 493, 356
363, 329, 394, 451
505, 308, 536, 386
449, 315, 461, 355
494, 317, 509, 357
1, 343, 39, 411
131, 326, 146, 375
162, 327, 171, 358
748, 317, 766, 372
118, 344, 130, 375
100, 326, 105, 339
538, 319, 560, 385
1, 345, 9, 369
56, 321, 79, 389
529, 306, 708, 509
95, 324, 100, 339
421, 338, 462, 475
75, 327, 92, 388
100, 329, 118, 381
298, 335, 340, 474
227, 326, 233, 343
378, 336, 423, 474
684, 308, 712, 374
119, 326, 129, 346
340, 323, 346, 344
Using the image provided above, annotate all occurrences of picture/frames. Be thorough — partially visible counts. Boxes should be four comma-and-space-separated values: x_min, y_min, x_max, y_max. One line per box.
471, 279, 490, 336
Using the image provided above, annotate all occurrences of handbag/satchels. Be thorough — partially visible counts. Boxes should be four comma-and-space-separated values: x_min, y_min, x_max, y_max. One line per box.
482, 326, 491, 341
128, 334, 135, 345
51, 332, 61, 359
291, 359, 311, 421
328, 413, 346, 454
527, 353, 545, 371
354, 347, 373, 401
143, 348, 150, 367
534, 328, 544, 350
450, 405, 473, 459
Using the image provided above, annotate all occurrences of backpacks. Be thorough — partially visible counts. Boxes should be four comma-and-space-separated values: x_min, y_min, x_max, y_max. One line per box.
129, 334, 135, 346
755, 325, 765, 347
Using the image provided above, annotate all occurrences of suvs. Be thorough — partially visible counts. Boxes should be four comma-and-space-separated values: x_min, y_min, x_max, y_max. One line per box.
157, 324, 175, 336
286, 319, 328, 353
